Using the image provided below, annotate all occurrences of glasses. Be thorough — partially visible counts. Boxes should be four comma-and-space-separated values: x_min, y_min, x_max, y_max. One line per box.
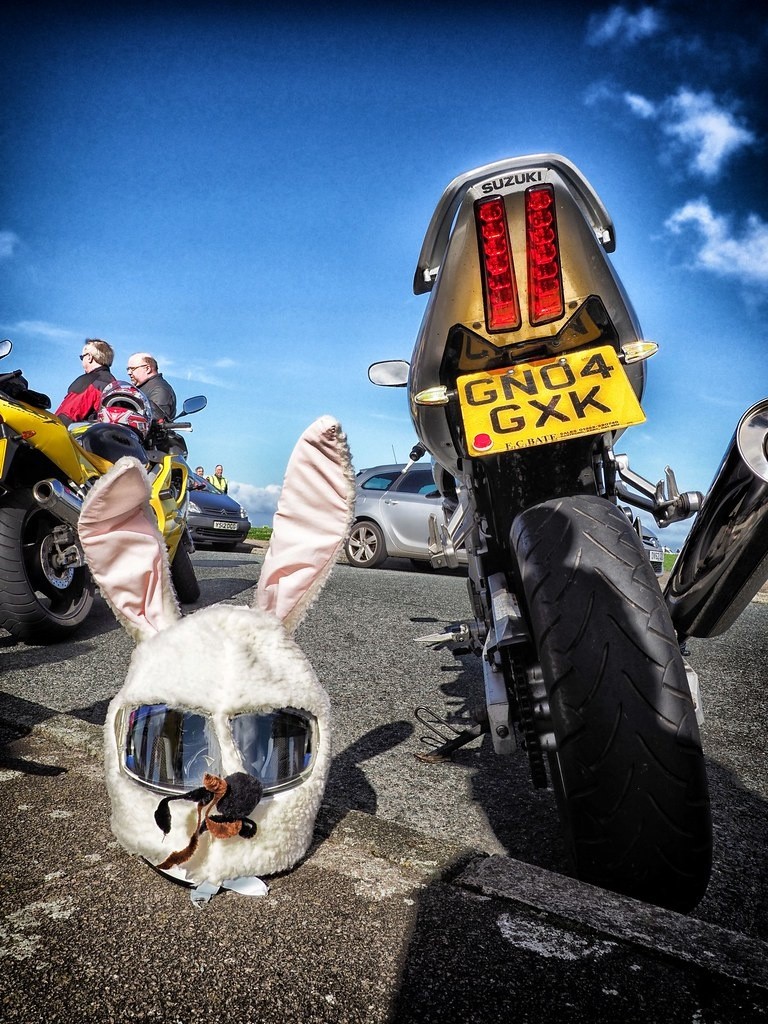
79, 352, 96, 360
114, 699, 321, 800
127, 365, 146, 372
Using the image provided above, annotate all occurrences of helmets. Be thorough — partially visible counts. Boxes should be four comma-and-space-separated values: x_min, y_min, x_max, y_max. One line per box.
98, 380, 152, 445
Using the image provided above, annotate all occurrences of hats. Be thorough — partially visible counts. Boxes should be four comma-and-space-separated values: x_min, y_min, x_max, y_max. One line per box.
76, 416, 358, 907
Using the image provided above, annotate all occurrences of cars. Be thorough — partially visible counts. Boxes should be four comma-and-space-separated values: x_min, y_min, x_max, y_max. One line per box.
184, 473, 251, 550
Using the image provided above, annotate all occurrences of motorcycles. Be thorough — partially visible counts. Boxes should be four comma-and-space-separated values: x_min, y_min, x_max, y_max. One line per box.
367, 153, 767, 915
0, 395, 209, 646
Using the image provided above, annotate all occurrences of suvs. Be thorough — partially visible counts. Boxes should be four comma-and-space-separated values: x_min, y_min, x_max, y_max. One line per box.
345, 463, 665, 578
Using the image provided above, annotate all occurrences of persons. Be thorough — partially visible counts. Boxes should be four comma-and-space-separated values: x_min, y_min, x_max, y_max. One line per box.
195, 466, 206, 490
206, 465, 228, 494
127, 353, 177, 420
54, 339, 117, 425
